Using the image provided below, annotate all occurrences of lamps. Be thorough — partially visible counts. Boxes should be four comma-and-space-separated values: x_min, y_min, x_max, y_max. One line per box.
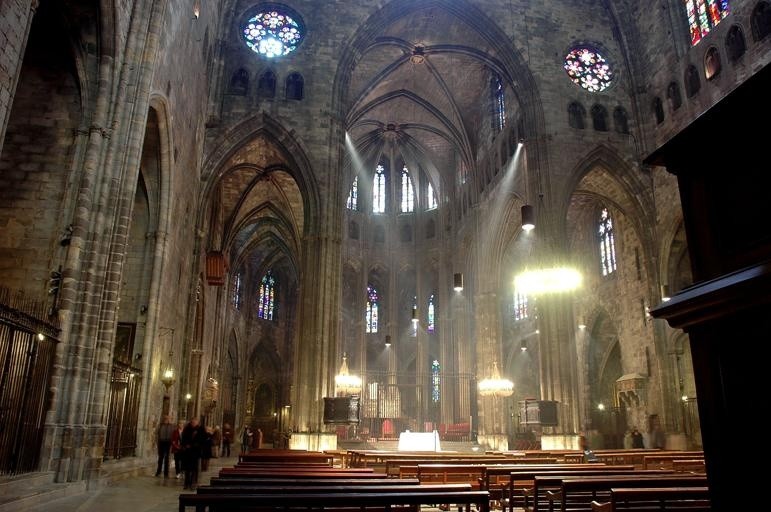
453, 56, 464, 294
385, 118, 391, 347
520, 339, 526, 350
578, 316, 586, 328
521, 70, 537, 232
411, 51, 420, 323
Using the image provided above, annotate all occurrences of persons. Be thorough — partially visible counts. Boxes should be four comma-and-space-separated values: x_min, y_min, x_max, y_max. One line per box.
630, 429, 645, 448
151, 414, 263, 491
577, 431, 592, 450
645, 414, 667, 449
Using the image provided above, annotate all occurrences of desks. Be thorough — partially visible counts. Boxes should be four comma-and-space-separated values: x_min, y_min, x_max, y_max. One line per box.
178, 447, 706, 512
